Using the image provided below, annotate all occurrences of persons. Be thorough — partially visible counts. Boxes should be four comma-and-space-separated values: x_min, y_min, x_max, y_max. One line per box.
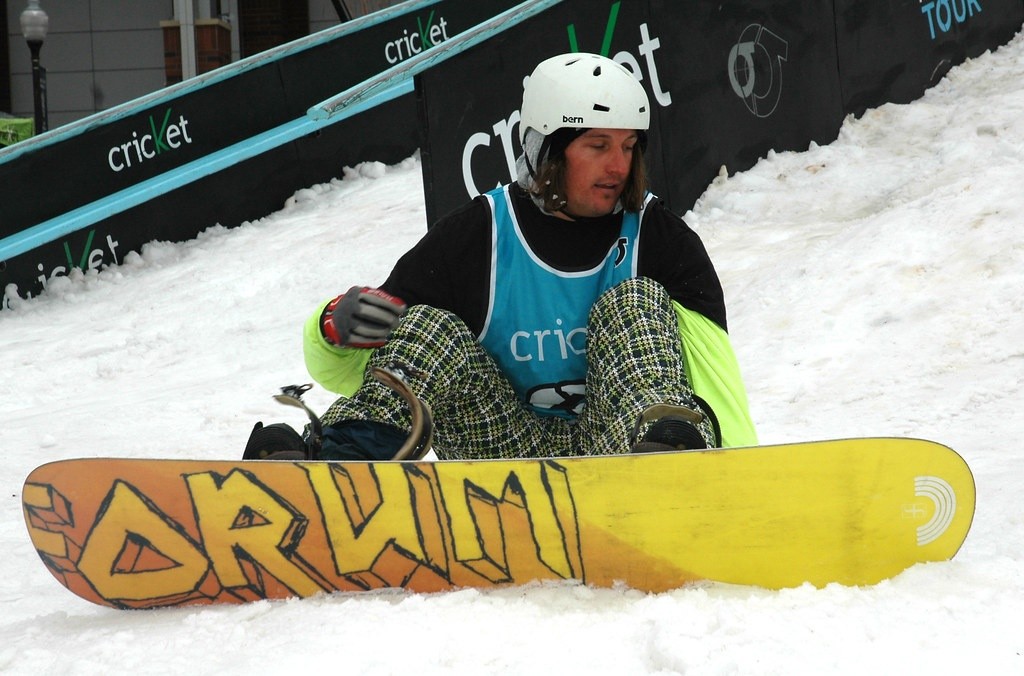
241, 54, 758, 459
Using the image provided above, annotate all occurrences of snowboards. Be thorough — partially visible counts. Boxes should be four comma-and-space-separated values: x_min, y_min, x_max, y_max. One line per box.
19, 432, 980, 611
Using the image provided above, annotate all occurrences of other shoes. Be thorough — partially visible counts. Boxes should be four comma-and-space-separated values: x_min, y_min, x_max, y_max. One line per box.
634, 416, 709, 454
242, 421, 310, 461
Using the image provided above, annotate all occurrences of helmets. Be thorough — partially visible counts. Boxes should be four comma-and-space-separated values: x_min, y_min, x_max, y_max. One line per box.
519, 52, 650, 145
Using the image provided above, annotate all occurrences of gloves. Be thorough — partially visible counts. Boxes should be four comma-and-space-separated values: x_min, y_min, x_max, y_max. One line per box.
321, 285, 405, 348
